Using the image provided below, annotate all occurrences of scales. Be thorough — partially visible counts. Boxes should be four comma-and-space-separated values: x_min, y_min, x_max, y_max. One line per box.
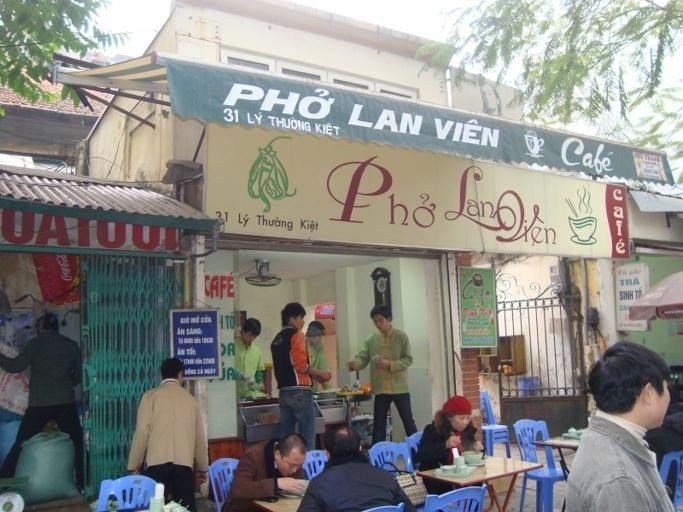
0, 475, 28, 511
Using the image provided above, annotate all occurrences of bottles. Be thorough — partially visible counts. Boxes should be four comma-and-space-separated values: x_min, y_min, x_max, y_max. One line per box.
106, 492, 117, 511
254, 370, 265, 393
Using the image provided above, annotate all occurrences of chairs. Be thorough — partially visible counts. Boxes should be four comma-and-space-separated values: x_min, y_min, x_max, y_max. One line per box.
479, 389, 511, 457
513, 419, 570, 512
303, 449, 328, 481
368, 441, 413, 478
658, 451, 683, 511
209, 457, 240, 512
96, 474, 161, 512
415, 484, 488, 512
362, 502, 405, 512
404, 430, 423, 468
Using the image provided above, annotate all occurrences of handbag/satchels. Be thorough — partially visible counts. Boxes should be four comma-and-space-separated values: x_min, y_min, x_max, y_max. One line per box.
381, 461, 429, 505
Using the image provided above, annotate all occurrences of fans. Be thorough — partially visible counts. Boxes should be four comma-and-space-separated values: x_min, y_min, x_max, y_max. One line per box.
244, 257, 281, 287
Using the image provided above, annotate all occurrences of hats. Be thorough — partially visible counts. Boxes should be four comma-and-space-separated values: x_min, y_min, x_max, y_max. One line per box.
306, 320, 326, 336
443, 396, 472, 415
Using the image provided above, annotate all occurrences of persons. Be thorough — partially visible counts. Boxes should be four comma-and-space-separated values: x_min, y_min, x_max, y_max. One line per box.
564, 339, 678, 512
125, 357, 209, 511
414, 394, 488, 511
302, 320, 338, 393
0, 310, 94, 500
231, 317, 267, 400
641, 382, 682, 502
219, 430, 307, 510
270, 300, 332, 456
291, 425, 417, 512
345, 305, 418, 460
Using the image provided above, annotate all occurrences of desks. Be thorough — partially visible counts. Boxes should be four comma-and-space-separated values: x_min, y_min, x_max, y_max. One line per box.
529, 429, 580, 512
417, 456, 544, 512
250, 491, 304, 512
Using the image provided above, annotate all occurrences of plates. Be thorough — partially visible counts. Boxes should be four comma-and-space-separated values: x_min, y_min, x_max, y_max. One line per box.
280, 493, 301, 498
437, 449, 486, 477
317, 389, 337, 405
562, 431, 582, 438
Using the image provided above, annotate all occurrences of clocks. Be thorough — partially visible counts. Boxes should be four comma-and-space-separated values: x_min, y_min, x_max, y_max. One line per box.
368, 267, 394, 328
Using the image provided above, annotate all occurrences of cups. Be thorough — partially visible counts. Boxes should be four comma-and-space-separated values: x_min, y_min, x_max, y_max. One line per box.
149, 496, 164, 512
297, 479, 309, 496
567, 427, 576, 436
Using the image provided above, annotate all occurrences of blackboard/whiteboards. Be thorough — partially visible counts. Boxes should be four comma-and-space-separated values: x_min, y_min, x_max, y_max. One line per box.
169, 308, 223, 381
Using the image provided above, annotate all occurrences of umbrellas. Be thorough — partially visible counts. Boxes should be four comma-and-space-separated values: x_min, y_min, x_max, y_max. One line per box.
629, 270, 682, 321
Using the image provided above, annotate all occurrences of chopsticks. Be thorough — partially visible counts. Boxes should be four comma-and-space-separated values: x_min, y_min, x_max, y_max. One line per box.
449, 432, 464, 453
